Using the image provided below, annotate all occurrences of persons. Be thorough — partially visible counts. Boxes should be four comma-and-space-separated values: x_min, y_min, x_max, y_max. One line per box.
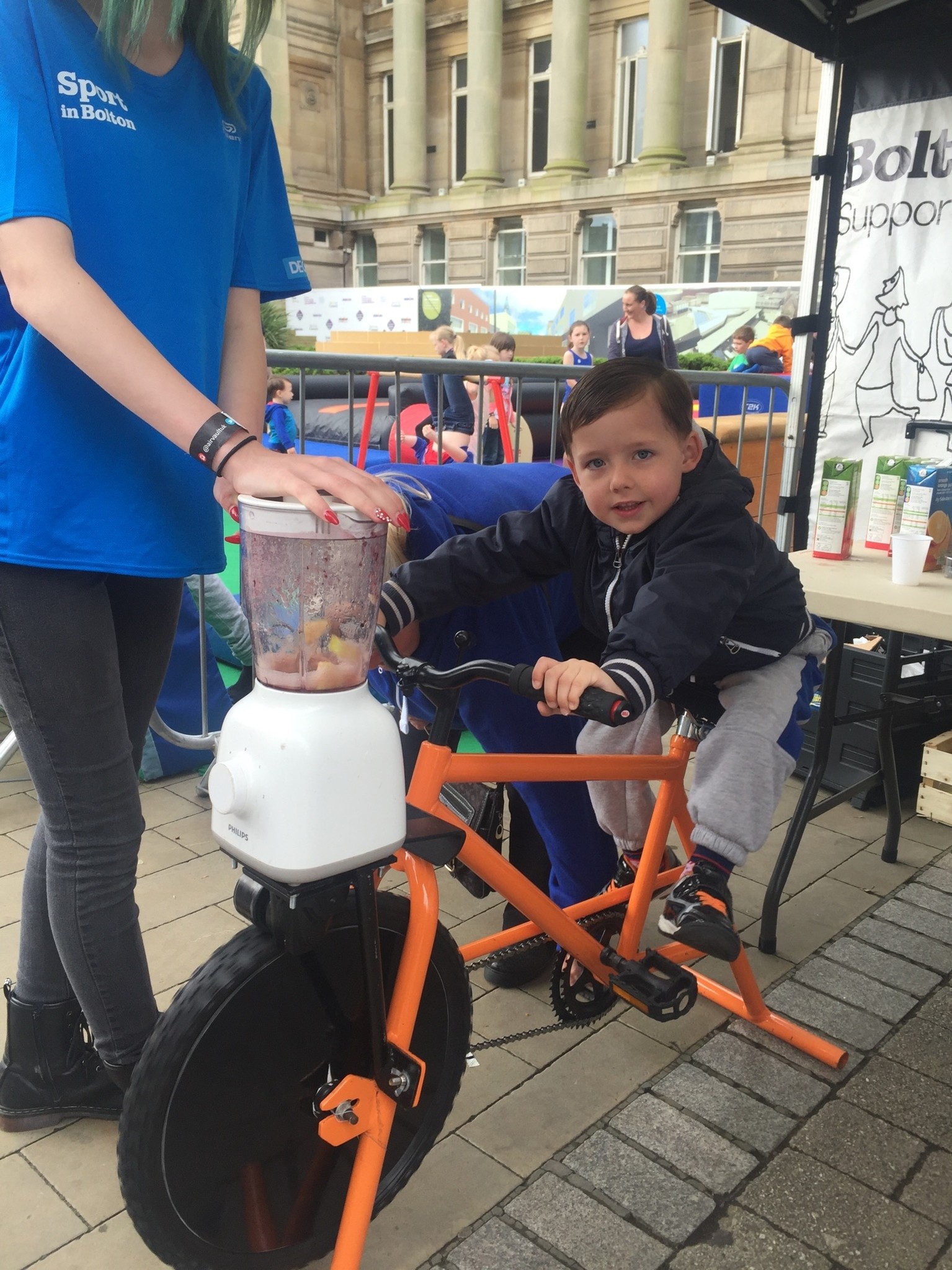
0, 0, 414, 1133
395, 324, 516, 466
731, 315, 796, 373
606, 284, 681, 370
263, 376, 299, 455
560, 320, 593, 468
137, 574, 253, 784
726, 325, 754, 372
315, 356, 841, 999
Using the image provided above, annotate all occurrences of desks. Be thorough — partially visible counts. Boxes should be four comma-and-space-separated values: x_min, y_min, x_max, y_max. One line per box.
757, 549, 952, 955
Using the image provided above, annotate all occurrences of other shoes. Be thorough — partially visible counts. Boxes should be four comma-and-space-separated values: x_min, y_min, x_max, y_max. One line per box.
483, 941, 555, 989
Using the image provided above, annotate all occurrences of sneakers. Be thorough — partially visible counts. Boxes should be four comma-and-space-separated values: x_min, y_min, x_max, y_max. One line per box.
594, 851, 682, 910
657, 861, 741, 963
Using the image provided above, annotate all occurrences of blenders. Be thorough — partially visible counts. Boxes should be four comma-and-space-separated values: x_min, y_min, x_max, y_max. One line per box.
207, 492, 406, 884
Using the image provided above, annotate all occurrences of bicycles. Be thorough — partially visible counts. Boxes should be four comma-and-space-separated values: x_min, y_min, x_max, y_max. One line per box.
118, 625, 851, 1270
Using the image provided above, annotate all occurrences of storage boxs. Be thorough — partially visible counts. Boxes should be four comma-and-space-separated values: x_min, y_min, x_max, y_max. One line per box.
794, 624, 952, 827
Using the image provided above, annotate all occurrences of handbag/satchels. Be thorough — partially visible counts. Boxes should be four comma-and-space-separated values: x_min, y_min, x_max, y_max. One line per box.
379, 727, 505, 898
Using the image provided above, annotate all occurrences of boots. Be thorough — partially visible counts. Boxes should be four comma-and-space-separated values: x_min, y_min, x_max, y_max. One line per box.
95, 1010, 165, 1093
0, 978, 124, 1132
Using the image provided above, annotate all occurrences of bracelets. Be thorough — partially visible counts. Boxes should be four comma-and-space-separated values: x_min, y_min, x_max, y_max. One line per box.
217, 436, 257, 477
189, 411, 249, 472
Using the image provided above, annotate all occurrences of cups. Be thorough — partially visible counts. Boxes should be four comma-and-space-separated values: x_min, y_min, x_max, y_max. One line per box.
890, 533, 934, 587
